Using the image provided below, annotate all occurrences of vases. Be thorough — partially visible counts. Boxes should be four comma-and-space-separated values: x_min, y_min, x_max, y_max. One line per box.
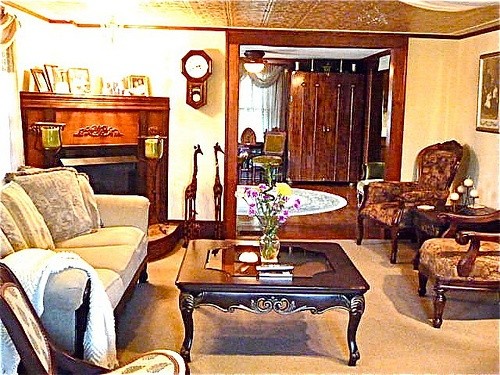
257, 226, 282, 265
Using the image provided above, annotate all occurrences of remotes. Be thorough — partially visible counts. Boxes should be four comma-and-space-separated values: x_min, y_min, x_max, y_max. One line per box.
255, 265, 294, 270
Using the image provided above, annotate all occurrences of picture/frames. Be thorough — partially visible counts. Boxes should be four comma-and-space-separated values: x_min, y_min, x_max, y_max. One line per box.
475, 50, 500, 135
127, 73, 151, 98
29, 67, 51, 93
44, 64, 94, 96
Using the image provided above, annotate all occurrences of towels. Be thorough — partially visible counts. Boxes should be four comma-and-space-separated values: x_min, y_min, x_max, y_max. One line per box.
1, 244, 122, 374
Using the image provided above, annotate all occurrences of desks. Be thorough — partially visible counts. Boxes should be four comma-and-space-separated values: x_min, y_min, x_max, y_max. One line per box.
238, 141, 265, 170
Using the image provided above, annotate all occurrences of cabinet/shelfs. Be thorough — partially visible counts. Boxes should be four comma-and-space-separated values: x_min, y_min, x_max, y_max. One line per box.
19, 89, 183, 264
285, 70, 368, 186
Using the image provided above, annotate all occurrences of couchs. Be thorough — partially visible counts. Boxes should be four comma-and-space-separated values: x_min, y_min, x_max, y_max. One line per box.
1, 164, 152, 360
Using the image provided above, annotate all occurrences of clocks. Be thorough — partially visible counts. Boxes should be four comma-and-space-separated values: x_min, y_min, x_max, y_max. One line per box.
180, 49, 211, 111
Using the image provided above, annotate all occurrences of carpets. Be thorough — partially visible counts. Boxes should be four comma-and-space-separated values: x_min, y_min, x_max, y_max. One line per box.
118, 238, 500, 375
236, 185, 349, 217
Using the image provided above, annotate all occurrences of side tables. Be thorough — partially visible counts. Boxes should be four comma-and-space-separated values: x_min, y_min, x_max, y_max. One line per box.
408, 204, 500, 271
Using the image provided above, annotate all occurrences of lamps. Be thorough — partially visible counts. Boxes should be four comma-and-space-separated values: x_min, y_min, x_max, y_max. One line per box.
28, 120, 68, 169
242, 49, 267, 75
449, 176, 481, 215
135, 134, 170, 235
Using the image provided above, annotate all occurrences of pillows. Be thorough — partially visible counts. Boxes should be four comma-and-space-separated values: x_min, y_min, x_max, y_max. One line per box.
1, 178, 56, 253
12, 164, 94, 244
74, 171, 106, 237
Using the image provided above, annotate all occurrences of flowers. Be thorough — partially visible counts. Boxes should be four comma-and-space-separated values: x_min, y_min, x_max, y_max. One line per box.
234, 181, 303, 225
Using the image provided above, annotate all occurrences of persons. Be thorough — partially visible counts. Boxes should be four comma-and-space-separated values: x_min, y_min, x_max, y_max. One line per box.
133, 79, 145, 95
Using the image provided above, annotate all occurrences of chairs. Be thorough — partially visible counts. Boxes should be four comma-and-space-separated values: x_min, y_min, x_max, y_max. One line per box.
1, 262, 192, 374
354, 139, 465, 265
416, 209, 500, 330
251, 126, 288, 184
240, 127, 257, 144
238, 157, 244, 175
